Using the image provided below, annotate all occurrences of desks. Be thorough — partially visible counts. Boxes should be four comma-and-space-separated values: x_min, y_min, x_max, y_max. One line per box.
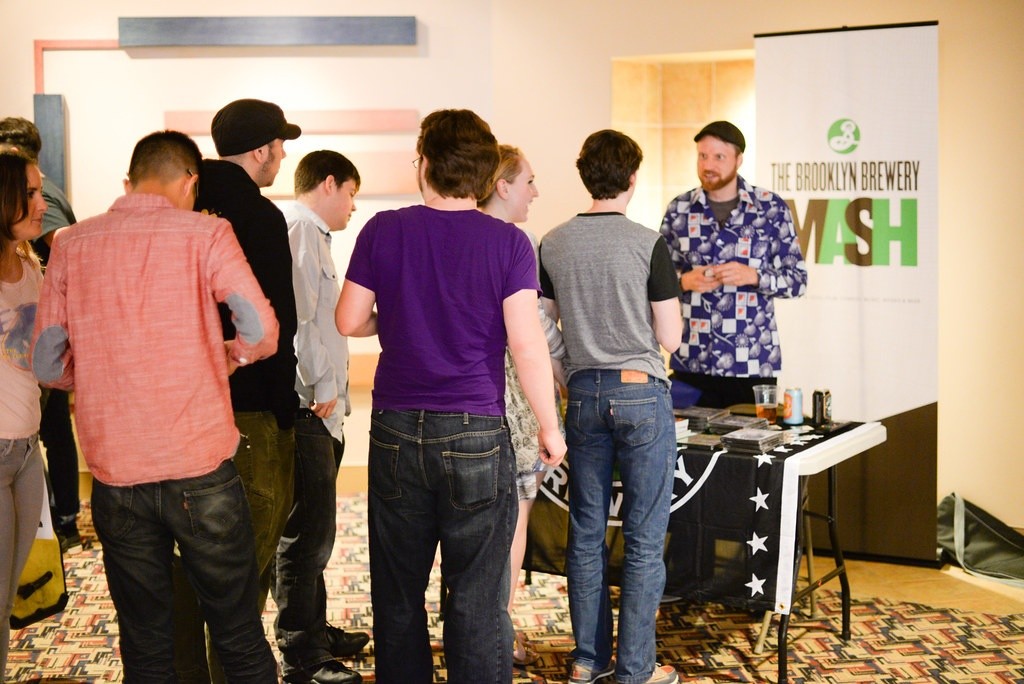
439, 415, 888, 684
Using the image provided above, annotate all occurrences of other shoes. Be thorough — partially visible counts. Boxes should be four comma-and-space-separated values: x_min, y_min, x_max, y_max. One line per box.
54, 521, 84, 552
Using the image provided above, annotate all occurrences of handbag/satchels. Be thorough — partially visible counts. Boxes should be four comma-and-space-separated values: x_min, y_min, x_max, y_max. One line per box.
13, 539, 67, 629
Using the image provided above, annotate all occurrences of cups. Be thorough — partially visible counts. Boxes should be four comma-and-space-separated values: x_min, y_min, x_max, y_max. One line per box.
753, 384, 781, 424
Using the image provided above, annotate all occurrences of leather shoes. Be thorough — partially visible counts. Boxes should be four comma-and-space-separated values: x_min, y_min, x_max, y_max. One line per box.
284, 647, 363, 684
319, 622, 370, 656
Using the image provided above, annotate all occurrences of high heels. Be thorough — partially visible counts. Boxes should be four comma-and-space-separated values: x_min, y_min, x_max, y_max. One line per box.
513, 634, 540, 665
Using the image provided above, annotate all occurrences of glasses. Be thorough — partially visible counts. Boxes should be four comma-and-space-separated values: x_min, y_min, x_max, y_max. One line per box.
413, 157, 424, 167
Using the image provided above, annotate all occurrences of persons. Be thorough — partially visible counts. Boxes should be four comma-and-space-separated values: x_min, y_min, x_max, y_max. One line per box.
27, 131, 279, 684
336, 110, 568, 684
539, 127, 684, 684
1, 143, 49, 684
477, 145, 567, 669
657, 121, 807, 604
0, 115, 85, 557
187, 97, 300, 684
273, 150, 369, 684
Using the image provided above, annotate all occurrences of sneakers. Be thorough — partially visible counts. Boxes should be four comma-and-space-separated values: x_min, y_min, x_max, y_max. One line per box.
616, 663, 679, 683
566, 657, 615, 683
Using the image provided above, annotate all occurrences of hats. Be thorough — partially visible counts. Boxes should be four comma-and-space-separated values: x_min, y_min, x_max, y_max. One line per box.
694, 121, 747, 154
211, 98, 302, 159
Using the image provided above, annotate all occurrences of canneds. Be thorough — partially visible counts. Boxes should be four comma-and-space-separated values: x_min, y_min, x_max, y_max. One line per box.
784, 387, 804, 425
813, 389, 832, 425
